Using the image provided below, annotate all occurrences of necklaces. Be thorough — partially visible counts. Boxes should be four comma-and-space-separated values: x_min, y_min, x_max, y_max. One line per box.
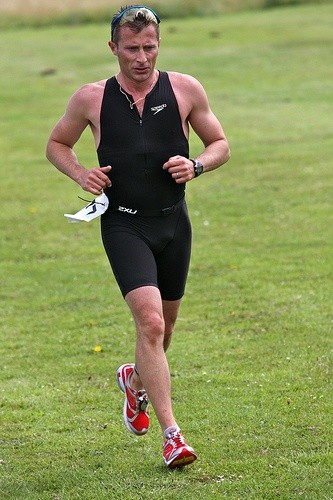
115, 72, 157, 110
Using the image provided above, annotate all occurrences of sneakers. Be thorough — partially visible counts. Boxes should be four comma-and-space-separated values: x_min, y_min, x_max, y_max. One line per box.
163, 426, 197, 467
116, 364, 150, 436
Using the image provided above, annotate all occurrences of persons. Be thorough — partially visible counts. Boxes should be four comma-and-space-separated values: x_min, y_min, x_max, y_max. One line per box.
46, 4, 231, 469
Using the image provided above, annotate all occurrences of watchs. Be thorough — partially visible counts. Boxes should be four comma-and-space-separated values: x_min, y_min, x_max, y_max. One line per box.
189, 158, 203, 178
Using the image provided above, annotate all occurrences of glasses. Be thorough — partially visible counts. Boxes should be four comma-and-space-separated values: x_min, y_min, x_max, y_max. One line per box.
111, 5, 161, 43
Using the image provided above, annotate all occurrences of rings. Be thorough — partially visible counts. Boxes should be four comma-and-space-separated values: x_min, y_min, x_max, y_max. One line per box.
176, 172, 179, 179
